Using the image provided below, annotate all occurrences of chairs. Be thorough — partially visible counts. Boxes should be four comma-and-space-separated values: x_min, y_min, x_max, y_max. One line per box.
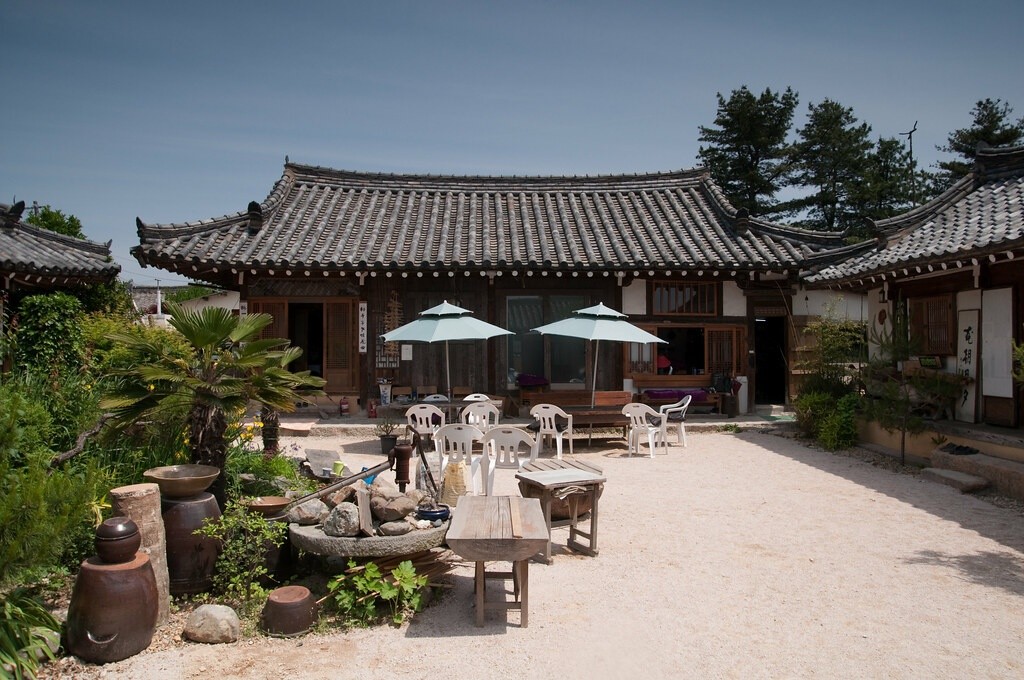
453, 387, 473, 399
621, 402, 669, 459
656, 394, 691, 446
391, 386, 413, 403
417, 385, 437, 401
527, 403, 574, 460
461, 402, 501, 456
405, 404, 446, 462
431, 423, 496, 496
481, 427, 538, 497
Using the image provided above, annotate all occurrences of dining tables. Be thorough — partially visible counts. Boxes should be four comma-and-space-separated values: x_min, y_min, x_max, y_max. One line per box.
559, 410, 631, 442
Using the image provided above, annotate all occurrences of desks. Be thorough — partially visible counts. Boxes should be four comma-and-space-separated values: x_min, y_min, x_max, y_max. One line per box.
519, 389, 632, 407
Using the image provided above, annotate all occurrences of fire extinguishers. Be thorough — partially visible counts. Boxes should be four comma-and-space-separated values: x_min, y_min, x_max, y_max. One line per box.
337, 396, 353, 419
367, 397, 378, 418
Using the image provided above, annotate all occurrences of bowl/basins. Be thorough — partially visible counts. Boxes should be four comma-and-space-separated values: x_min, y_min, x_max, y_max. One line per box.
238, 496, 292, 516
143, 463, 221, 499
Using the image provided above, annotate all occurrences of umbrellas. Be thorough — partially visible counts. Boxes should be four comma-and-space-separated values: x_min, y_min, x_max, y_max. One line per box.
529, 301, 669, 407
379, 299, 517, 424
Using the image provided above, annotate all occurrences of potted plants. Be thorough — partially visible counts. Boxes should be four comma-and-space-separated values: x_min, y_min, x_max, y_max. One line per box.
374, 403, 400, 454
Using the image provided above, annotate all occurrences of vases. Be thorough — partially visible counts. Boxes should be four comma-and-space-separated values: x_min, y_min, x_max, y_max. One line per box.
418, 504, 450, 523
722, 393, 738, 419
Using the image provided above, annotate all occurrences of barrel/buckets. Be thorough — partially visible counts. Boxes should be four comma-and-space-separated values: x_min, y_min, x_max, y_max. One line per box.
69, 553, 159, 665
94, 517, 140, 563
243, 512, 293, 589
259, 584, 319, 638
159, 491, 224, 596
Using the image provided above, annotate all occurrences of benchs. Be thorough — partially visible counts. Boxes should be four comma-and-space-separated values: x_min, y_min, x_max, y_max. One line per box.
642, 389, 722, 414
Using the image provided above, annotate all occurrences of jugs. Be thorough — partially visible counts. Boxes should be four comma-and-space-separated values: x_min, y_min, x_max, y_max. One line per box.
333, 461, 347, 477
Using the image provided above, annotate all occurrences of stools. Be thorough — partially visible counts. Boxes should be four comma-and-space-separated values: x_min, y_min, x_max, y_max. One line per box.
514, 468, 607, 566
445, 495, 549, 629
